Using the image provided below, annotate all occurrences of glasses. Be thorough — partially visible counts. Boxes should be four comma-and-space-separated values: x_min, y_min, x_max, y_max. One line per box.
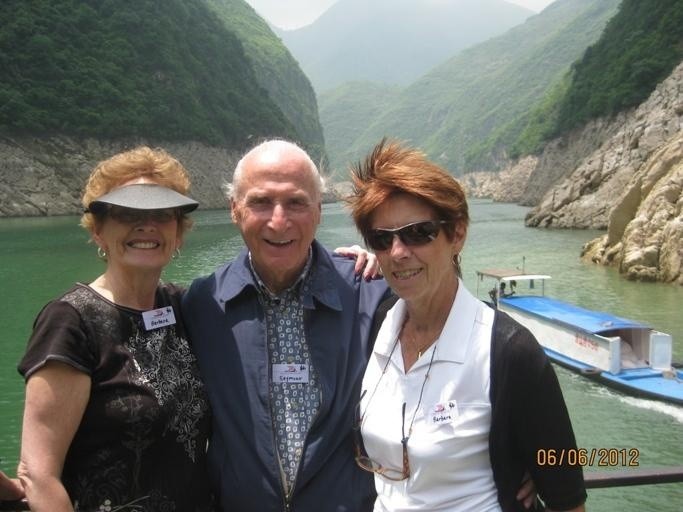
354, 389, 410, 482
366, 221, 454, 251
102, 206, 173, 224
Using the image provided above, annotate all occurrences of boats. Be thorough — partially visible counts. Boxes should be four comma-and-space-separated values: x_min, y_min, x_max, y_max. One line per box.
475, 256, 683, 409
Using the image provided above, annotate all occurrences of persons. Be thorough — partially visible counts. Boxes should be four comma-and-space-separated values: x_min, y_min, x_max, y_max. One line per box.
348, 137, 588, 512
1, 472, 25, 502
18, 145, 382, 512
181, 138, 538, 512
489, 283, 515, 305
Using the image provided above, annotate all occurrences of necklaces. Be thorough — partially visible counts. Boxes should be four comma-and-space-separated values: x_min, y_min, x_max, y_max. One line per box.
404, 314, 442, 359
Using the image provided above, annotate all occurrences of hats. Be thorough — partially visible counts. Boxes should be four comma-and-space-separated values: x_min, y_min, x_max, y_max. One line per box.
89, 183, 200, 214
